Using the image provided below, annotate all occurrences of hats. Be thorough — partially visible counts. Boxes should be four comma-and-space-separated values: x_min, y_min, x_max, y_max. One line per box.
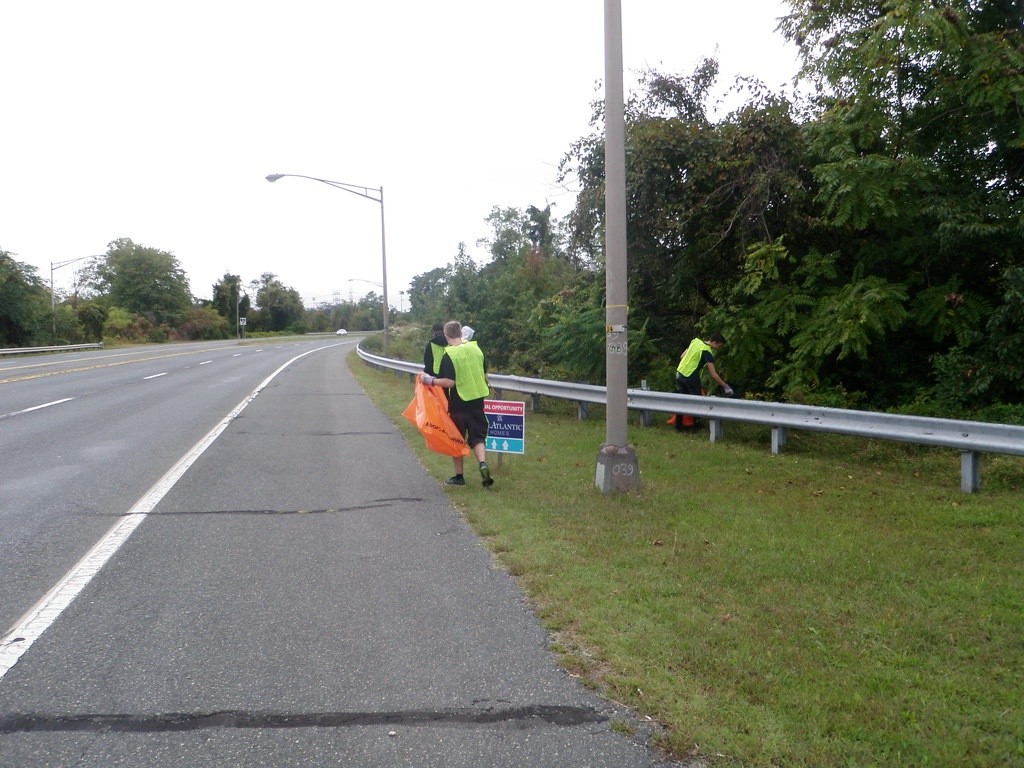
433, 324, 443, 331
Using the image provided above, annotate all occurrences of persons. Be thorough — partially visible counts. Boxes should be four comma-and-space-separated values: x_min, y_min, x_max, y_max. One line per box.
424, 324, 450, 400
675, 332, 734, 433
421, 321, 495, 487
460, 325, 474, 343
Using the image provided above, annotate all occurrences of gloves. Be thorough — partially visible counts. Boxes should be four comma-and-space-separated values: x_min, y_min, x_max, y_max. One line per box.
723, 384, 733, 395
421, 374, 435, 387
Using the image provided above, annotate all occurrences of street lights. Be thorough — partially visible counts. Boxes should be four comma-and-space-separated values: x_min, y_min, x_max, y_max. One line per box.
50, 254, 108, 335
265, 171, 390, 358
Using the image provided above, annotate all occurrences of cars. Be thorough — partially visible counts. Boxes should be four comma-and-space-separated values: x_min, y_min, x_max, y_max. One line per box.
336, 328, 347, 337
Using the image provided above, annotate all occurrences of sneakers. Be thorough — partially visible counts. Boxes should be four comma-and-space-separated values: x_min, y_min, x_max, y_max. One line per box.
479, 462, 494, 487
444, 477, 466, 487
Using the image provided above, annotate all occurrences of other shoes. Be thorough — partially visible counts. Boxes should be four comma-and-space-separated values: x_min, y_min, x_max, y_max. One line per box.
675, 424, 689, 433
693, 422, 703, 431
